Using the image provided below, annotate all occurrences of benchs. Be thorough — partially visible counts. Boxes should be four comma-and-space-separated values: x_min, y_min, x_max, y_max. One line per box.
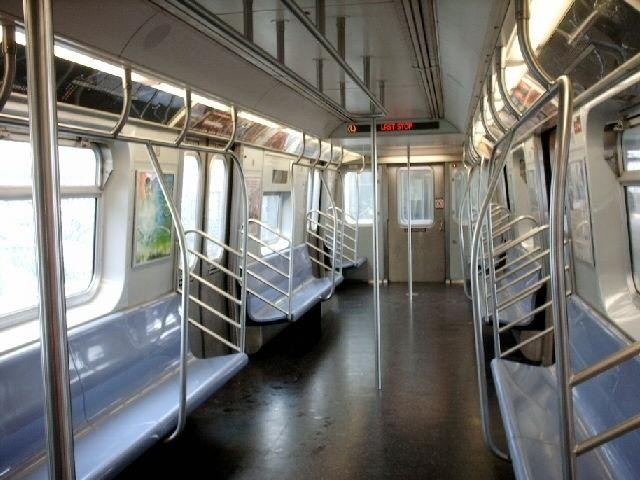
244, 240, 344, 324
489, 292, 640, 480
472, 242, 541, 326
326, 227, 368, 269
469, 229, 503, 270
0, 290, 248, 480
